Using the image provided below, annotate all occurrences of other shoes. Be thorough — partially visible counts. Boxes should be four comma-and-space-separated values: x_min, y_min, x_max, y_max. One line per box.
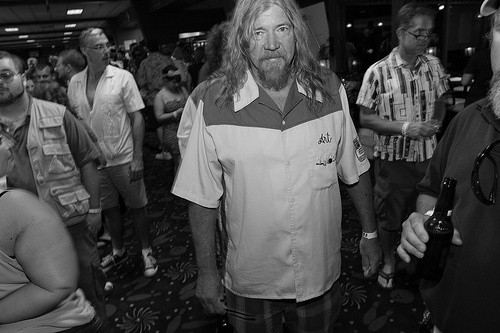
97, 234, 112, 247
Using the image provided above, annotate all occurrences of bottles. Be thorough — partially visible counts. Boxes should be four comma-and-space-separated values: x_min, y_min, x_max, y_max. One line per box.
414, 176, 457, 282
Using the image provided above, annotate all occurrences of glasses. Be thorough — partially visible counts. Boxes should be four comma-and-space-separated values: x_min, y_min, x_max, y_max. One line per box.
400, 27, 437, 42
0, 71, 22, 83
84, 40, 111, 51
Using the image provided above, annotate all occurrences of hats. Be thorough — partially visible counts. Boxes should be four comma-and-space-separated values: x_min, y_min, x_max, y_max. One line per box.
480, 0, 500, 16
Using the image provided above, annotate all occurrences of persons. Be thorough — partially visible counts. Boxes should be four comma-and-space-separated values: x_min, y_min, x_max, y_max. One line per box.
0, 0, 500, 333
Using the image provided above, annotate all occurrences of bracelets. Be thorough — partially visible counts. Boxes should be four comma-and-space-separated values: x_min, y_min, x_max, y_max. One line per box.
362, 230, 378, 239
402, 121, 411, 136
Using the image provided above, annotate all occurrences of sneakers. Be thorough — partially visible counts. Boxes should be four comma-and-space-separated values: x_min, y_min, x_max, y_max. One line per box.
100, 248, 128, 273
142, 249, 158, 276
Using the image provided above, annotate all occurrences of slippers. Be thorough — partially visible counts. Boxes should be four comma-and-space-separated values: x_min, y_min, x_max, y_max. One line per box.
378, 263, 395, 289
